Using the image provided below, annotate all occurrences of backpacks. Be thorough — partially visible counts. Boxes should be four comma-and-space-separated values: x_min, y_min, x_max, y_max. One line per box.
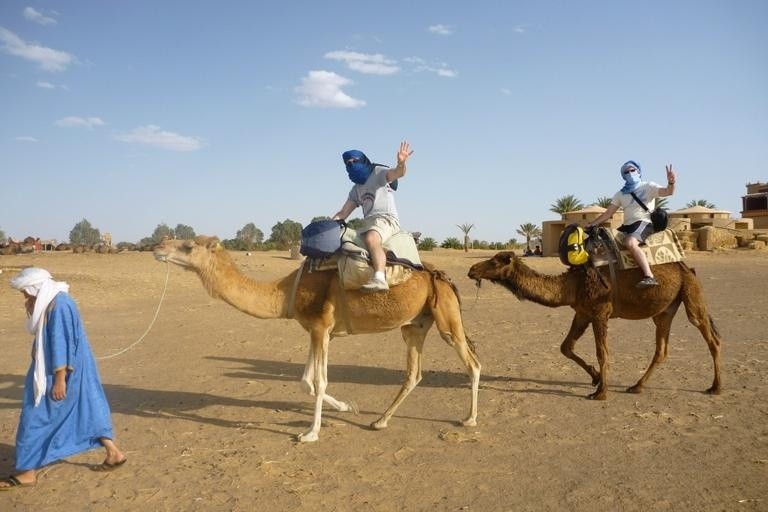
558, 223, 590, 266
300, 218, 346, 258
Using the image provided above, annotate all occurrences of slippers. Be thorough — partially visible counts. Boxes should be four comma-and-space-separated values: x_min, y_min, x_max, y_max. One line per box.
0, 475, 36, 490
95, 459, 125, 471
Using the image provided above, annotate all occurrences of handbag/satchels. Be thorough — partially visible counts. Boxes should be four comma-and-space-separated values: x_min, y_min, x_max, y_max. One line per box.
651, 206, 668, 231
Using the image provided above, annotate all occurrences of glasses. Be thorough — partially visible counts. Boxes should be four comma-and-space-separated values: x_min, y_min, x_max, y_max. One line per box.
346, 157, 361, 166
624, 168, 636, 173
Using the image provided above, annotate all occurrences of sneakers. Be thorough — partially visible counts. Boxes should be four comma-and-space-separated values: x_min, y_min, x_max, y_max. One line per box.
360, 278, 390, 294
635, 276, 659, 288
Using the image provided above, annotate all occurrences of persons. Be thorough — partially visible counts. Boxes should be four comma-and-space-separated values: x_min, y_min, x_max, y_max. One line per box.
327, 141, 415, 293
524, 246, 534, 256
585, 161, 675, 290
0, 267, 128, 490
522, 249, 526, 256
534, 246, 542, 255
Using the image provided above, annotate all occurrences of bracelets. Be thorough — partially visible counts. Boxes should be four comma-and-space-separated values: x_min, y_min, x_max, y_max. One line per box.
667, 181, 675, 186
